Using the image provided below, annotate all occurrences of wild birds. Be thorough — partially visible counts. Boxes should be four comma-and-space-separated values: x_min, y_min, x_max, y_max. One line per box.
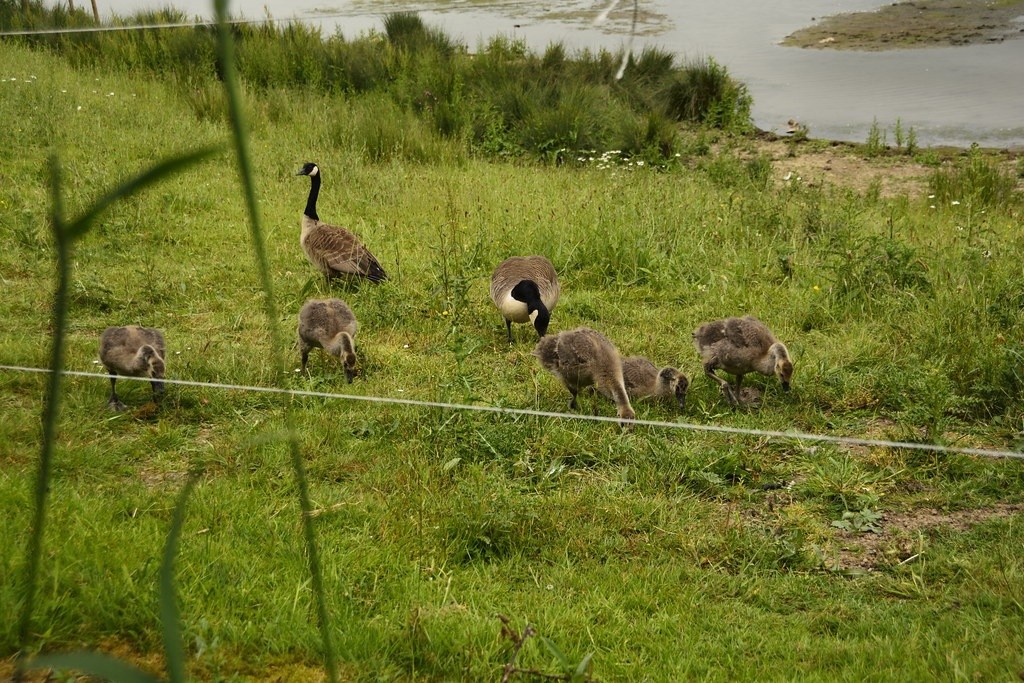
691, 315, 794, 415
294, 162, 388, 286
98, 324, 167, 412
298, 298, 358, 385
530, 326, 689, 435
490, 254, 561, 344
